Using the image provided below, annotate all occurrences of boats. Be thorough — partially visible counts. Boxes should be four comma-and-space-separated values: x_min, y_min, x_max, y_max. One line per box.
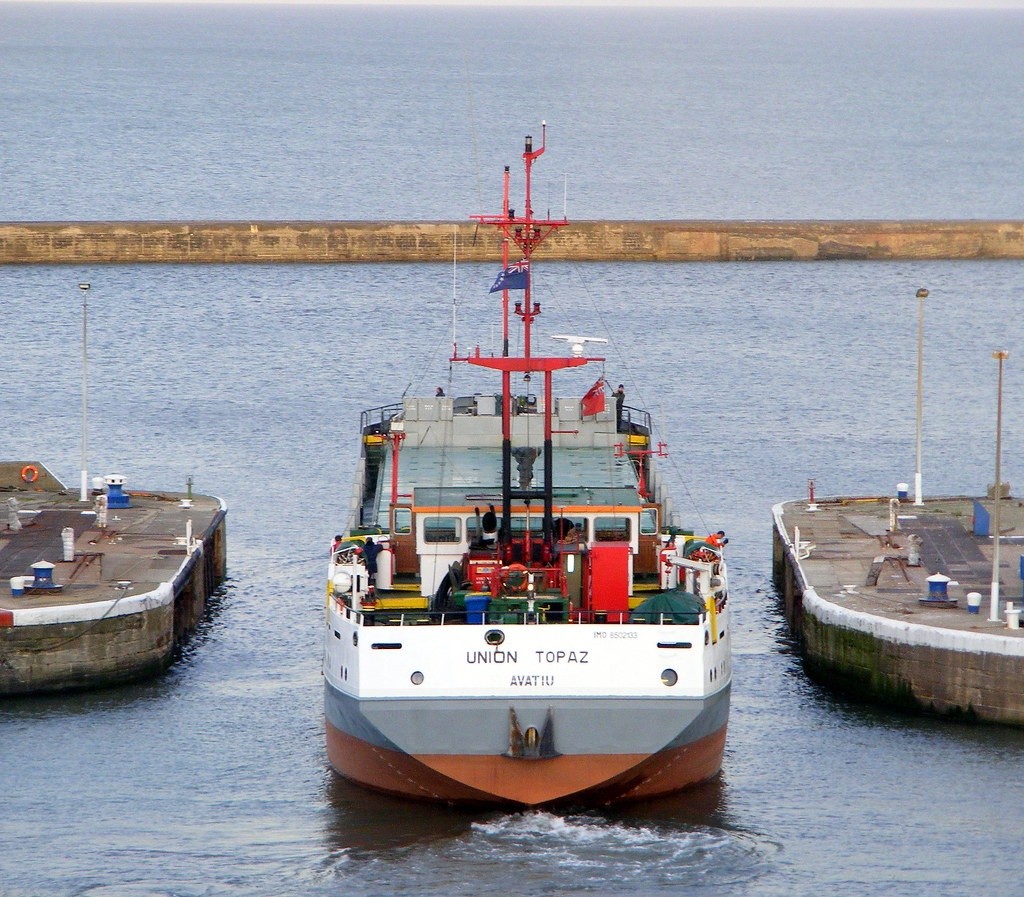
321, 116, 730, 815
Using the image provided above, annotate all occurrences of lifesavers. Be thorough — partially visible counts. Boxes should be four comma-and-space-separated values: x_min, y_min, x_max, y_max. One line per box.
505, 564, 529, 593
21, 465, 38, 483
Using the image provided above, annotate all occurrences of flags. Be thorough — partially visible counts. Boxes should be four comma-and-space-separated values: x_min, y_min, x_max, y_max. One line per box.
581, 374, 605, 416
488, 257, 528, 293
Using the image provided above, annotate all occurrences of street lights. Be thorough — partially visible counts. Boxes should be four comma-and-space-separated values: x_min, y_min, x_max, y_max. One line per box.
78, 281, 93, 502
911, 287, 930, 506
985, 349, 1012, 623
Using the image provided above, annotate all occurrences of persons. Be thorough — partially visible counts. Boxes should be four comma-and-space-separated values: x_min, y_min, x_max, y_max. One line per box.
330, 535, 343, 554
362, 537, 383, 579
705, 530, 728, 548
611, 384, 626, 432
